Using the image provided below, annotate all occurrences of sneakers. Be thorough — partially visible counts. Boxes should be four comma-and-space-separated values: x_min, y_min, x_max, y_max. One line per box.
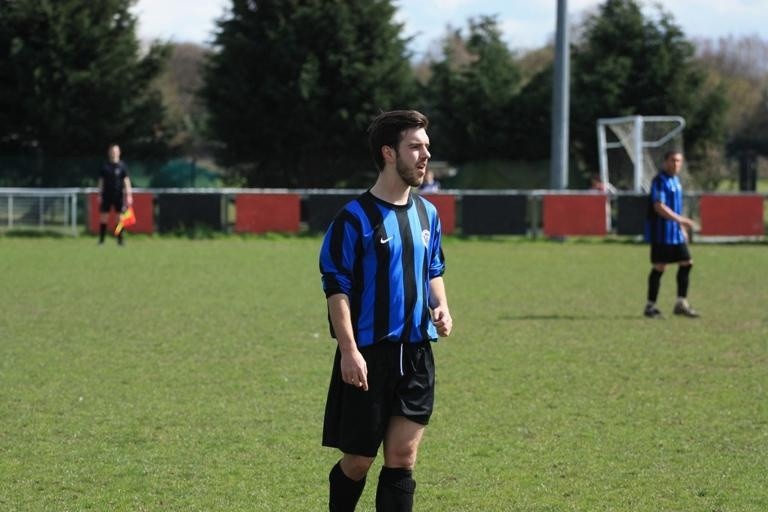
644, 304, 665, 319
673, 301, 700, 318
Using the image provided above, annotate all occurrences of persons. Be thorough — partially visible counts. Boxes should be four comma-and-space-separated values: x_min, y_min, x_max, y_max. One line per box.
590, 175, 617, 229
643, 149, 702, 318
318, 110, 454, 512
419, 170, 442, 193
96, 144, 135, 246
